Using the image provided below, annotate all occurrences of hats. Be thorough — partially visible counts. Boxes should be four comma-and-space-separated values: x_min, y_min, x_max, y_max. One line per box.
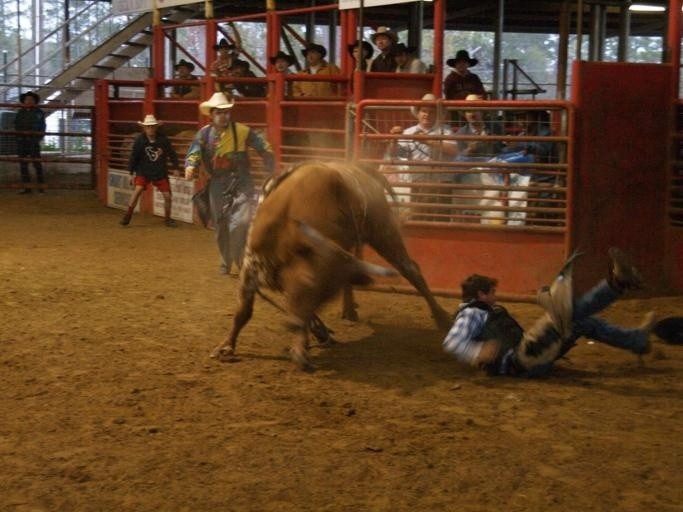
509, 99, 549, 127
299, 42, 328, 61
407, 92, 451, 122
226, 58, 251, 72
212, 37, 237, 52
136, 113, 162, 129
345, 39, 375, 61
172, 58, 196, 75
197, 90, 237, 116
455, 93, 495, 119
388, 42, 416, 58
444, 48, 478, 69
367, 25, 401, 49
17, 90, 40, 105
267, 50, 295, 67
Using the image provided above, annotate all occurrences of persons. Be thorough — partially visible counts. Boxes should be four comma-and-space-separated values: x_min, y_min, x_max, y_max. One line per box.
14, 91, 45, 194
169, 60, 201, 99
269, 44, 342, 99
184, 92, 275, 274
443, 49, 487, 101
387, 93, 460, 222
443, 247, 656, 379
450, 94, 559, 226
346, 26, 426, 75
121, 114, 179, 227
210, 38, 267, 96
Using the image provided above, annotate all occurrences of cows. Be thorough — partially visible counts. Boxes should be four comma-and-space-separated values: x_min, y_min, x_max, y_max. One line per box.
208, 159, 455, 373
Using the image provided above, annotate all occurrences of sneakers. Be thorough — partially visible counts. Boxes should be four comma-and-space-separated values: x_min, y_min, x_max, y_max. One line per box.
14, 187, 49, 196
606, 245, 648, 293
163, 217, 178, 228
219, 262, 232, 274
635, 308, 659, 356
118, 216, 130, 226
